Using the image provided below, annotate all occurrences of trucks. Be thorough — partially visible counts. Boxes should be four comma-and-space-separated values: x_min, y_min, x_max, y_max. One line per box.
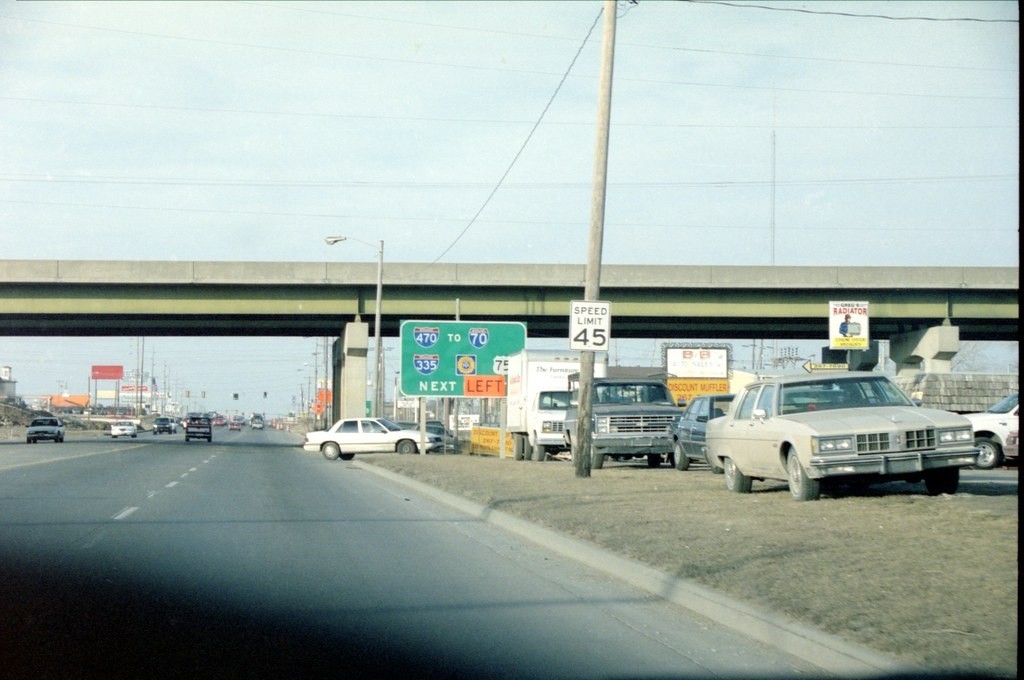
508, 348, 609, 463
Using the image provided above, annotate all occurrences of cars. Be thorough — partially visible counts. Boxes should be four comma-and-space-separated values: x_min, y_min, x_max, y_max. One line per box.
707, 369, 980, 500
305, 416, 442, 460
213, 412, 266, 432
566, 374, 685, 471
184, 417, 213, 442
110, 421, 137, 438
398, 421, 459, 455
25, 417, 65, 443
672, 394, 735, 473
151, 413, 214, 434
961, 391, 1018, 468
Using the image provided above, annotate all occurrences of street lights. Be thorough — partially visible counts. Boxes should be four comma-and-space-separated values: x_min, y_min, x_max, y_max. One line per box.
289, 335, 327, 417
326, 232, 386, 418
